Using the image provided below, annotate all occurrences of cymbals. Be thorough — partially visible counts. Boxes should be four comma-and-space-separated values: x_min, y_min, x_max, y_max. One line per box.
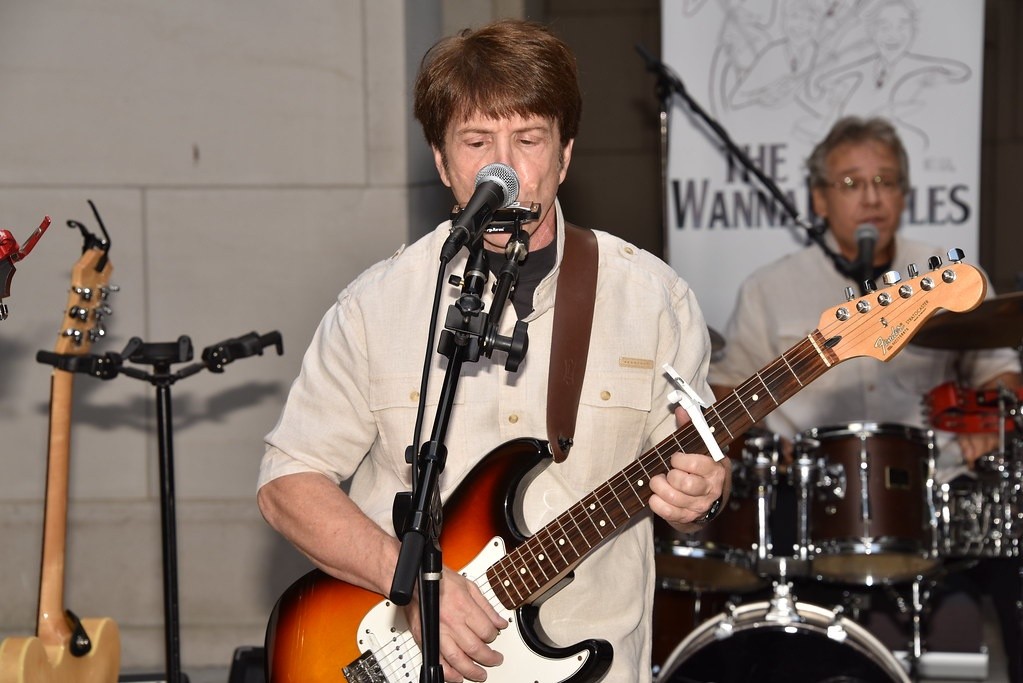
920, 381, 1022, 434
908, 292, 1023, 349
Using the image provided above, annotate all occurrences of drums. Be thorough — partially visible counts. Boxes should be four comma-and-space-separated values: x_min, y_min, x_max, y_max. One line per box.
652, 426, 784, 595
788, 420, 940, 586
654, 596, 912, 683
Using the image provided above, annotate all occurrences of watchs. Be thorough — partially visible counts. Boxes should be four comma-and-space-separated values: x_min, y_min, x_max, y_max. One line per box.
686, 489, 723, 524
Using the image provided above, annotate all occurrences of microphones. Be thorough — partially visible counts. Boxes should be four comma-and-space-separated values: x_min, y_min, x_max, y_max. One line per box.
439, 162, 520, 262
853, 223, 879, 286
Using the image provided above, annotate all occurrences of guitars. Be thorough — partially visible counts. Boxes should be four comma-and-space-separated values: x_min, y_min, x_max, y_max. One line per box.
0, 200, 123, 683
263, 248, 987, 682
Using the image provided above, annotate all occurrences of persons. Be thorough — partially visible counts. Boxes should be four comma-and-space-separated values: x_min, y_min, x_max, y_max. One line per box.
708, 115, 1022, 473
257, 21, 733, 683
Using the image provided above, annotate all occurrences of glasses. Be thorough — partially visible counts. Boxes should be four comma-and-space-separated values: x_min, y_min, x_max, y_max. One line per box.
822, 174, 904, 192
